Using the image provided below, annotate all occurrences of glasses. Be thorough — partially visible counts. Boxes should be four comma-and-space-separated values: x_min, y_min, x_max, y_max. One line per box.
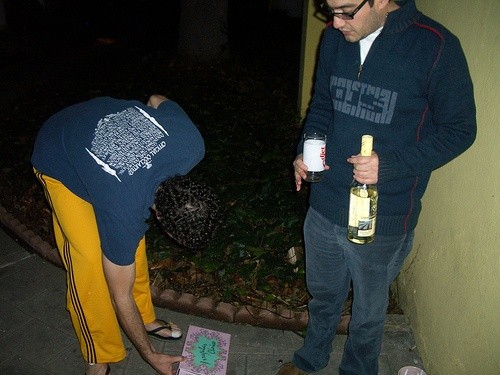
319, 0, 368, 20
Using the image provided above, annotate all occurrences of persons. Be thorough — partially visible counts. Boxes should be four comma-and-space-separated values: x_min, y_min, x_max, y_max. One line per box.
276, 0, 478, 375
31, 94, 223, 375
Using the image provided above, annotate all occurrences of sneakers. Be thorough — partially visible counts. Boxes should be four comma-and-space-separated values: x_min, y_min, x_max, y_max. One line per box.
277, 362, 328, 375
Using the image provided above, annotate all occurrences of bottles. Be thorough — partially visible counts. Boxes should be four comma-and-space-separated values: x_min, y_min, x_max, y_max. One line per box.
347, 134, 378, 245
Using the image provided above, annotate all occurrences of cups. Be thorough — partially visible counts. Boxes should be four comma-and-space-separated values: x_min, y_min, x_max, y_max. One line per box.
303, 133, 327, 182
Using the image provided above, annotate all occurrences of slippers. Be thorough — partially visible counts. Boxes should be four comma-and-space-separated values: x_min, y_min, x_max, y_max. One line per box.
147, 319, 183, 340
84, 365, 111, 375
